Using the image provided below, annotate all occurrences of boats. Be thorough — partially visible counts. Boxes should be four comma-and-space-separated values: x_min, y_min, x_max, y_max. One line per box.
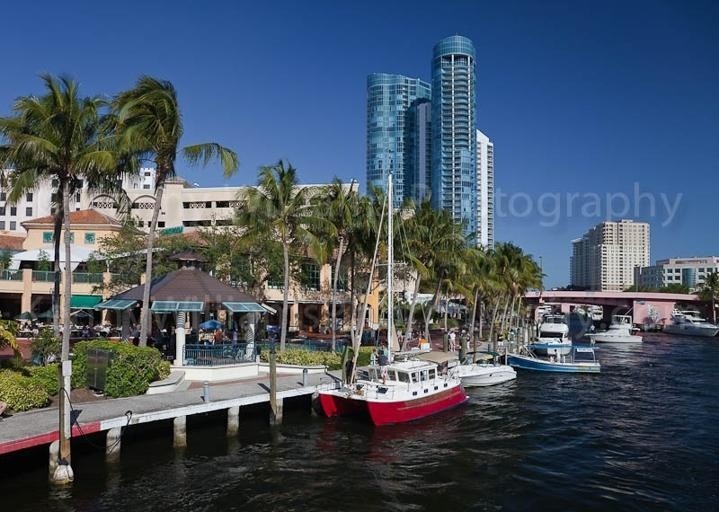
535, 304, 572, 356
663, 310, 719, 337
448, 351, 517, 388
572, 304, 644, 344
506, 342, 601, 375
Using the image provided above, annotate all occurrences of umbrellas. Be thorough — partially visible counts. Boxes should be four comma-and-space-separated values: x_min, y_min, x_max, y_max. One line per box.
69, 309, 93, 318
11, 311, 36, 320
35, 308, 54, 319
199, 320, 223, 329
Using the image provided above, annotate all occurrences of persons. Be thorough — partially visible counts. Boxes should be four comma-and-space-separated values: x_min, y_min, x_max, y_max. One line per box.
14, 319, 255, 358
374, 349, 388, 384
370, 349, 378, 365
416, 329, 472, 353
345, 356, 356, 381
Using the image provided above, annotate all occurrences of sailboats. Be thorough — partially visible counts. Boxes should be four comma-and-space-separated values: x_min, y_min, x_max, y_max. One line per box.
317, 172, 469, 427
